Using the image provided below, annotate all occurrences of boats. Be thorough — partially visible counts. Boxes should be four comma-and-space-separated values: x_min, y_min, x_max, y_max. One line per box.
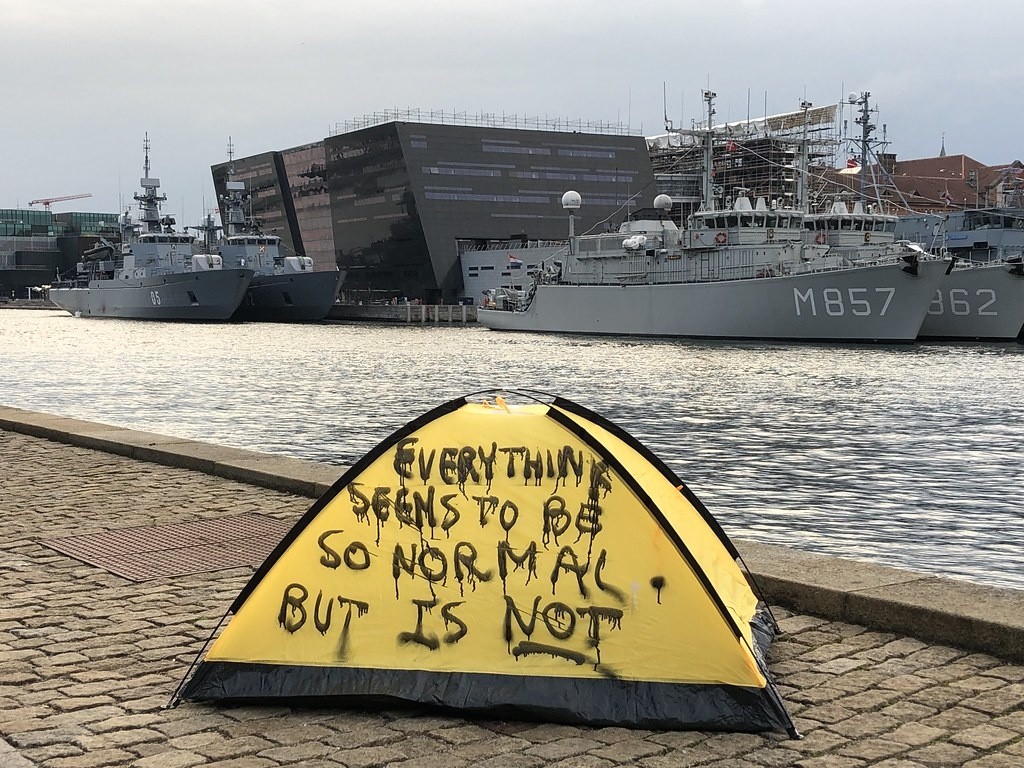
887, 202, 1022, 342
47, 131, 256, 325
475, 78, 959, 350
211, 136, 351, 325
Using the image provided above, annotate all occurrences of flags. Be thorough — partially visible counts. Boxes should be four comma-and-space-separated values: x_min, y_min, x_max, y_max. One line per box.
509, 253, 523, 266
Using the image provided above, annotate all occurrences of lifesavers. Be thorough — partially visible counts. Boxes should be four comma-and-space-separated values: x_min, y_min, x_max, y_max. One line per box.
715, 231, 728, 244
816, 232, 827, 245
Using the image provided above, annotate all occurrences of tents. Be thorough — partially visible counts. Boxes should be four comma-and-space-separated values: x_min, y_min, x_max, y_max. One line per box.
165, 385, 804, 740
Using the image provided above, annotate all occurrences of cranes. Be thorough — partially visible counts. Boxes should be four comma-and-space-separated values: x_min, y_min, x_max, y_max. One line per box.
27, 193, 92, 211
